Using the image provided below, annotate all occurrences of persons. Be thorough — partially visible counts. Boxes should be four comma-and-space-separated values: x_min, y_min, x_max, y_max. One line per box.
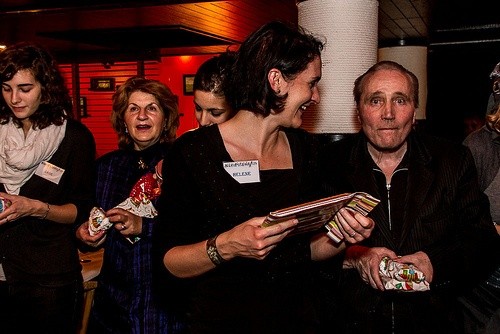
329, 60, 500, 334
73, 76, 181, 334
153, 19, 376, 334
0, 40, 96, 334
187, 51, 238, 133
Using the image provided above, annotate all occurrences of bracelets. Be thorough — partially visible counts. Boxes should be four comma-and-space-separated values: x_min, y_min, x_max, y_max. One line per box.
205, 234, 229, 267
38, 202, 50, 220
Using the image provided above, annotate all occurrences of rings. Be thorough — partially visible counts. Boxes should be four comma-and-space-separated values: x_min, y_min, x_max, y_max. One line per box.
350, 231, 356, 237
120, 223, 128, 230
